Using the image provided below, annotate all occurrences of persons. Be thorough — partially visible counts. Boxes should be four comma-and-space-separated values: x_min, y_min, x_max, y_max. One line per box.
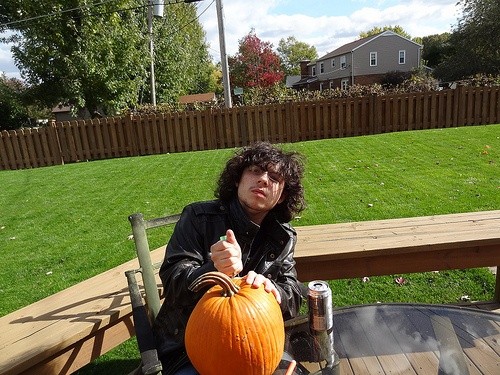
154, 141, 308, 375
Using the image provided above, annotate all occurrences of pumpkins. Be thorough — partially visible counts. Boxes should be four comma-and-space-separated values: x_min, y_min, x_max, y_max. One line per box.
185, 271, 285, 375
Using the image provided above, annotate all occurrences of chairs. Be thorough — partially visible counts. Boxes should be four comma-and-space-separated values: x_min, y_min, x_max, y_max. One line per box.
125, 213, 183, 375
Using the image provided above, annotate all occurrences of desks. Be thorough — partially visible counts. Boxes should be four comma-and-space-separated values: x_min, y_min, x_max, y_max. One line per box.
165, 303, 500, 375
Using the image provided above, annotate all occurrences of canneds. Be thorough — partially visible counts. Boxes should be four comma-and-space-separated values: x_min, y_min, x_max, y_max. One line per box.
307, 280, 333, 331
